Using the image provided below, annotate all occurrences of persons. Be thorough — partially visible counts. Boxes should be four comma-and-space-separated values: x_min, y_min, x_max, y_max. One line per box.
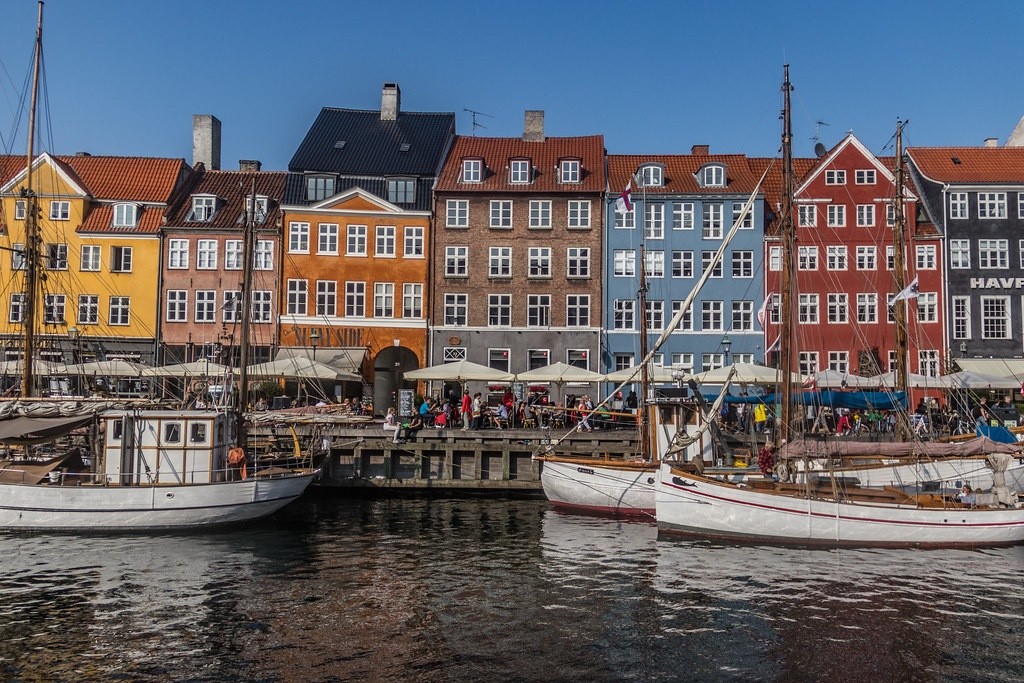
617, 389, 622, 401
594, 400, 612, 430
954, 485, 977, 508
575, 394, 592, 433
419, 391, 460, 429
728, 396, 1024, 437
400, 406, 423, 444
384, 407, 401, 443
517, 401, 539, 430
542, 402, 564, 431
625, 391, 638, 409
491, 402, 509, 431
758, 442, 775, 480
460, 390, 472, 431
472, 392, 484, 431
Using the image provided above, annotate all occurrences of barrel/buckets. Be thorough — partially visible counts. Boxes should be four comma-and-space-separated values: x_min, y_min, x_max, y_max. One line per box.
49, 471, 60, 483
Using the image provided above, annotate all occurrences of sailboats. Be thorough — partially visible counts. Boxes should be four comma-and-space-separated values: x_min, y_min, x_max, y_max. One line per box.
0, 0, 365, 537
531, 62, 1024, 549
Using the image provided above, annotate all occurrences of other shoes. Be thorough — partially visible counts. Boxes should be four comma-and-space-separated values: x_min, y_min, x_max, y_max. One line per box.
461, 428, 469, 431
496, 425, 502, 429
393, 440, 399, 443
399, 440, 406, 444
587, 429, 592, 432
423, 423, 432, 429
835, 433, 843, 437
576, 428, 583, 432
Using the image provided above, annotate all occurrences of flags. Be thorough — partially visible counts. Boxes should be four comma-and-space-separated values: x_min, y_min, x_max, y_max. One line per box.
888, 276, 921, 307
758, 292, 773, 331
616, 180, 633, 216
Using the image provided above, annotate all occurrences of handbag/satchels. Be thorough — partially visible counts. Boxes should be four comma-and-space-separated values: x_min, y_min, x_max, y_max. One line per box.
577, 412, 583, 421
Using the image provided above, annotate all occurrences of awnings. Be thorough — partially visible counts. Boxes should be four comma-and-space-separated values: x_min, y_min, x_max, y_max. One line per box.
954, 358, 1024, 391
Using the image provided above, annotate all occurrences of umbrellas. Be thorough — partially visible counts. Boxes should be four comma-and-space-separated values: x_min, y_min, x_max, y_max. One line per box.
2, 356, 360, 413
519, 361, 604, 402
608, 363, 1024, 391
402, 359, 515, 390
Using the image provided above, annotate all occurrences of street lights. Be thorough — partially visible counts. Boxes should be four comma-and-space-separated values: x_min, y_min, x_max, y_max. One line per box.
721, 335, 732, 430
67, 326, 80, 364
309, 329, 321, 406
